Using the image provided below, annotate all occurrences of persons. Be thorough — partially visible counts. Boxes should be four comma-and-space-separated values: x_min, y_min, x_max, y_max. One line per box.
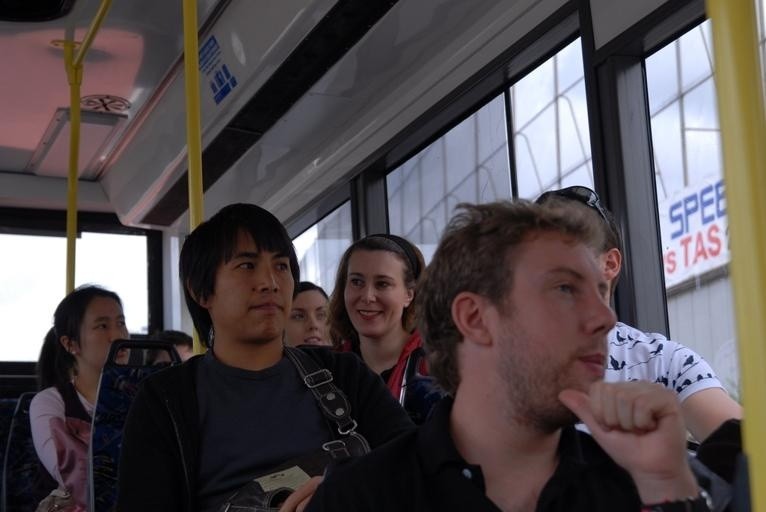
28, 286, 130, 509
535, 184, 744, 442
119, 201, 431, 511
308, 199, 711, 511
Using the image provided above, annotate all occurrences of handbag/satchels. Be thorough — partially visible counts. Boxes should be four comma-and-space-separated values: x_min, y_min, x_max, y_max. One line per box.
51, 414, 91, 507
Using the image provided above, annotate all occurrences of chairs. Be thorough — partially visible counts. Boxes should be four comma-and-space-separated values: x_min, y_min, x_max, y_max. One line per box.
85, 338, 186, 510
399, 343, 443, 426
2, 392, 58, 510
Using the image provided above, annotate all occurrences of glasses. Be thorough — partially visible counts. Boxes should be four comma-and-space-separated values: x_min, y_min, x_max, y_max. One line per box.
535, 186, 615, 232
